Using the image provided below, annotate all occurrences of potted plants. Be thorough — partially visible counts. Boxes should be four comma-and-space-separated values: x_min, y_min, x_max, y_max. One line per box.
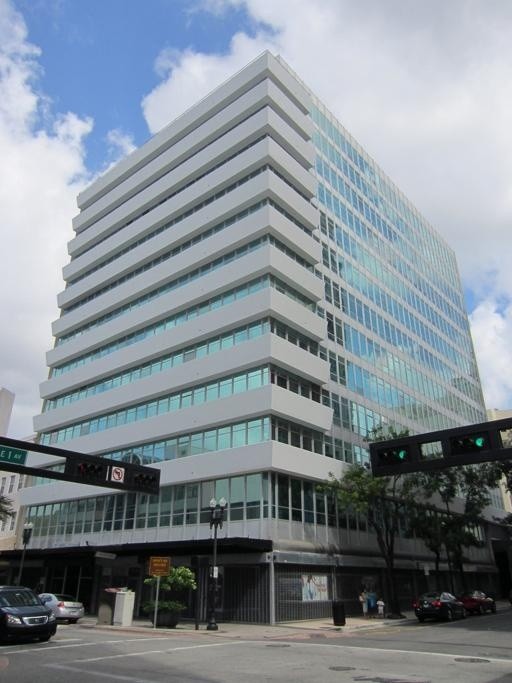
142, 565, 197, 628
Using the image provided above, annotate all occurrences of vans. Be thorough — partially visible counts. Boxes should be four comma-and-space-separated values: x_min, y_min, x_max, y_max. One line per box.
0, 585, 56, 644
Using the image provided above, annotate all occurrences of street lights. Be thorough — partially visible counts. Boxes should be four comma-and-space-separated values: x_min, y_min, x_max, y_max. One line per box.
206, 495, 226, 629
15, 521, 33, 583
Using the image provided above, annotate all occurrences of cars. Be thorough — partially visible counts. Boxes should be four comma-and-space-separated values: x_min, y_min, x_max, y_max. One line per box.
455, 588, 497, 615
413, 589, 467, 623
37, 591, 84, 623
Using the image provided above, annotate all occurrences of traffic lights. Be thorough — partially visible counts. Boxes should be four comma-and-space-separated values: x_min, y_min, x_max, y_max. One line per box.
73, 458, 102, 476
453, 429, 493, 452
133, 470, 158, 486
376, 446, 412, 465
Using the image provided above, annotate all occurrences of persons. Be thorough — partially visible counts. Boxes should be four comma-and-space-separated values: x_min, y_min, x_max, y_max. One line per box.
359, 589, 369, 617
367, 589, 379, 618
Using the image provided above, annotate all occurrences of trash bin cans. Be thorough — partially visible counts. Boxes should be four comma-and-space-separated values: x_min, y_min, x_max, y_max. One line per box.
332, 601, 345, 626
98, 588, 116, 625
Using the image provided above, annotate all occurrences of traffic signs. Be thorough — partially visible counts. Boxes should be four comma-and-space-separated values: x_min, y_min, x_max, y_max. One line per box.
0, 444, 29, 466
